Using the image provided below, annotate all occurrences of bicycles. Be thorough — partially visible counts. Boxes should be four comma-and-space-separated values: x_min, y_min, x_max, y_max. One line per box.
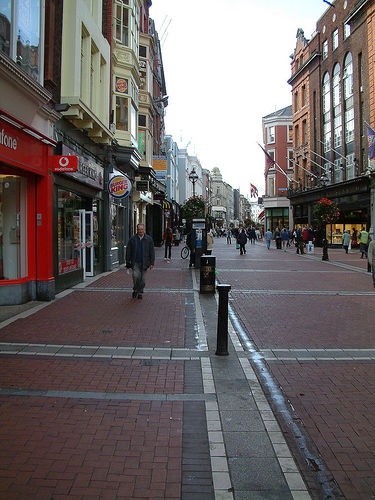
180, 245, 190, 259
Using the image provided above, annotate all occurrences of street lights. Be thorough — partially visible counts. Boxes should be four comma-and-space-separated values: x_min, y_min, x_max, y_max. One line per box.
188, 168, 199, 197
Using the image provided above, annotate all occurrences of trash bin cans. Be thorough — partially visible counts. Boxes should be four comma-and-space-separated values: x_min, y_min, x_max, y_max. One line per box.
276, 237, 282, 250
199, 255, 216, 296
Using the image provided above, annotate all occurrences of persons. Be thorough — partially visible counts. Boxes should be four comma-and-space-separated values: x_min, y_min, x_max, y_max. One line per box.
175, 228, 181, 247
264, 228, 272, 249
186, 228, 196, 267
358, 227, 372, 259
237, 228, 247, 255
274, 226, 313, 256
327, 231, 343, 244
212, 226, 264, 246
368, 239, 375, 288
205, 227, 214, 255
163, 227, 173, 261
342, 230, 350, 254
125, 224, 155, 299
352, 228, 357, 249
235, 228, 241, 249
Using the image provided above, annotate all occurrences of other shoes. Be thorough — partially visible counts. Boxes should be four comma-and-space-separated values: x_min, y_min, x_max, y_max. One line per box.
138, 293, 142, 299
132, 288, 137, 299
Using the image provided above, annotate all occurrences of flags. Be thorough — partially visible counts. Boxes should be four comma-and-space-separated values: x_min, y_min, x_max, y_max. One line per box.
250, 184, 258, 198
